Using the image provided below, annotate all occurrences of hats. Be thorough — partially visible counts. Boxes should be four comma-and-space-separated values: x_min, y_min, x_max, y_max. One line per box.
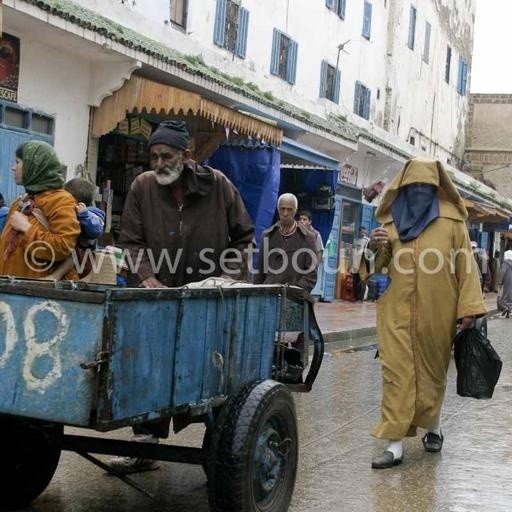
147, 120, 189, 151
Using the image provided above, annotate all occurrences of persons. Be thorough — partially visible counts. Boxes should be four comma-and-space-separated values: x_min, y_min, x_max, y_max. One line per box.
471, 240, 485, 286
252, 193, 319, 293
357, 158, 489, 469
482, 249, 493, 292
350, 226, 369, 304
105, 119, 258, 476
63, 178, 129, 287
491, 251, 501, 293
0, 139, 82, 283
298, 210, 324, 268
0, 192, 10, 233
497, 249, 512, 319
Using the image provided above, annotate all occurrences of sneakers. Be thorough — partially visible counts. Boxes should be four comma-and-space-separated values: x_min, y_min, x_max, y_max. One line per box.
109, 455, 161, 473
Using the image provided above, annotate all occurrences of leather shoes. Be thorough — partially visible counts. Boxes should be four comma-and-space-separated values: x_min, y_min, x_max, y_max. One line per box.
372, 451, 404, 469
422, 432, 444, 452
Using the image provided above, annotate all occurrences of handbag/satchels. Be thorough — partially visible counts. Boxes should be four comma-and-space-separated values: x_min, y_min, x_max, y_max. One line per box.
453, 321, 502, 399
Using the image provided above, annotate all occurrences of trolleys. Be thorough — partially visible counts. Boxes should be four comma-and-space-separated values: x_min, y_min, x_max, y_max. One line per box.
0, 276, 326, 512
